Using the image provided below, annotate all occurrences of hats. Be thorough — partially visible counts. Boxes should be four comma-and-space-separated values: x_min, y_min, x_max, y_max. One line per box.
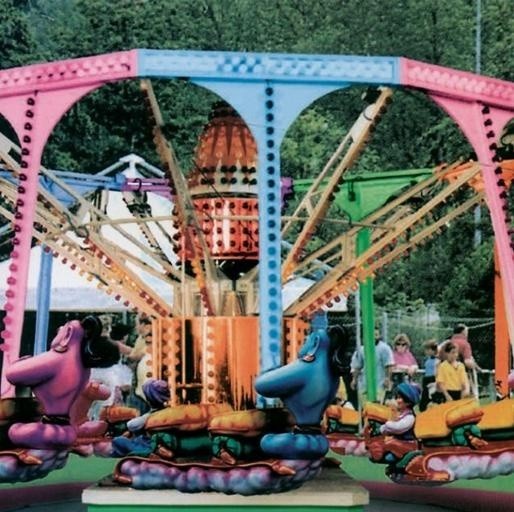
454, 324, 466, 333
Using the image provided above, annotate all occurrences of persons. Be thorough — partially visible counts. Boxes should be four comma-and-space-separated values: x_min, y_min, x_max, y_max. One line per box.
101, 311, 152, 416
251, 324, 357, 460
69, 379, 111, 437
350, 322, 476, 411
378, 379, 422, 439
112, 379, 172, 456
5, 314, 120, 450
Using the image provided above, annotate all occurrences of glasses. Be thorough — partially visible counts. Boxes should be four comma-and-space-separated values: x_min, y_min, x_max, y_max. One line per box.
396, 343, 406, 346
